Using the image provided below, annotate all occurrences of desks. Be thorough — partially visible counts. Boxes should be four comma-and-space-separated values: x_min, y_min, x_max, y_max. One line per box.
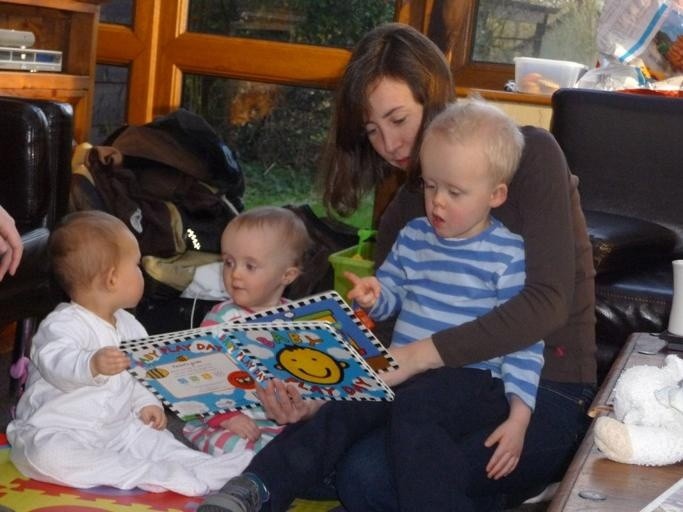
586, 330, 683, 416
547, 417, 680, 512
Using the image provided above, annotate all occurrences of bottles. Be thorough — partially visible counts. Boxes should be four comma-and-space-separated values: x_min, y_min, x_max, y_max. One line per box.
668, 257, 683, 335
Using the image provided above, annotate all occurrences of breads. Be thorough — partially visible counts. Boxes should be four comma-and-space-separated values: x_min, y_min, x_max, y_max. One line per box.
521, 73, 560, 95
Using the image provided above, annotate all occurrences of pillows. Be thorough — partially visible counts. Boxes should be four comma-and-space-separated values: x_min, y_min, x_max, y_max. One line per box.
568, 209, 673, 283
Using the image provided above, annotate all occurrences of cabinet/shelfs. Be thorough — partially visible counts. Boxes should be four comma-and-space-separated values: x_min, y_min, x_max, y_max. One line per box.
1, 0, 96, 166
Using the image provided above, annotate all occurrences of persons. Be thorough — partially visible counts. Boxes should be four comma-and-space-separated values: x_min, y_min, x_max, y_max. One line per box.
292, 21, 601, 511
5, 208, 257, 499
0, 207, 24, 280
182, 204, 310, 455
195, 100, 548, 511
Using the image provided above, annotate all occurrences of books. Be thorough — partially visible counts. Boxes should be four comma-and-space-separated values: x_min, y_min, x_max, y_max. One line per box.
118, 289, 401, 420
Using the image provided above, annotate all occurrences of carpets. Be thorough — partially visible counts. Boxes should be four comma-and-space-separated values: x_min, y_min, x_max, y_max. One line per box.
1, 420, 342, 509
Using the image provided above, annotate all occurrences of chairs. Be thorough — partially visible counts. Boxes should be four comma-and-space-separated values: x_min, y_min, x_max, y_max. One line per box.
539, 91, 683, 372
4, 91, 77, 340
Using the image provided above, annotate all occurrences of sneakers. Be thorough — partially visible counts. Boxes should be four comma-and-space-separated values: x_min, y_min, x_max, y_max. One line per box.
196, 476, 261, 512
